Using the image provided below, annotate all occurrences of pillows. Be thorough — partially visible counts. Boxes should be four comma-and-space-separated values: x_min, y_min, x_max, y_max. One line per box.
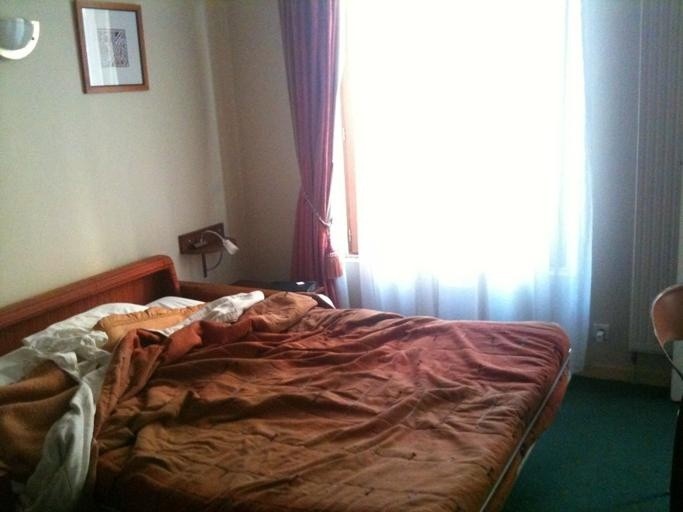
0, 290, 202, 387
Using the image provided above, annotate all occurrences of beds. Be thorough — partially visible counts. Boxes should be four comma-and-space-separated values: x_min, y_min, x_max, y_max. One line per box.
1, 254, 571, 508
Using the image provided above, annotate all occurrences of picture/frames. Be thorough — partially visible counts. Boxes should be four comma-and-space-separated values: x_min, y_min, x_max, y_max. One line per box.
71, 0, 151, 93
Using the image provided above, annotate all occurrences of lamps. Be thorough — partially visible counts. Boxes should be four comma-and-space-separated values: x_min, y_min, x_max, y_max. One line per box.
197, 227, 239, 278
1, 16, 41, 62
